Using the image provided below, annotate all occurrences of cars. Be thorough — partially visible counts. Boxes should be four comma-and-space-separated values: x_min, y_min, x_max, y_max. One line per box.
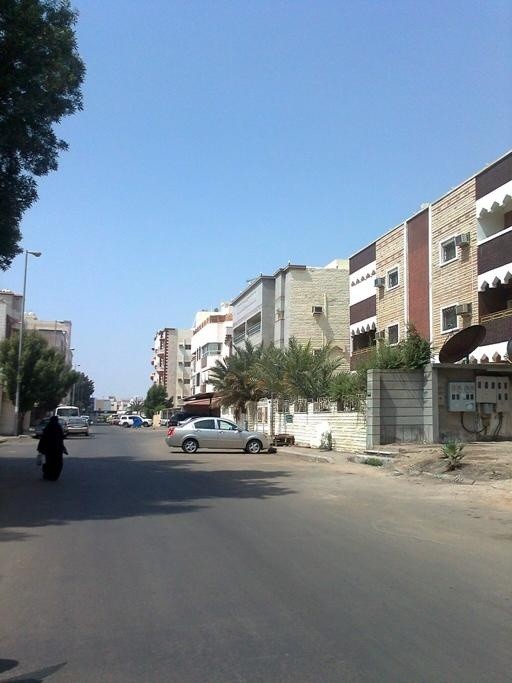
97, 414, 153, 428
160, 412, 268, 454
35, 406, 90, 439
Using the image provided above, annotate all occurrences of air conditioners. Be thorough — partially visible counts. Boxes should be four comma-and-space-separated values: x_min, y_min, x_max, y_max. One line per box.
312, 306, 323, 315
455, 234, 470, 247
375, 331, 385, 338
455, 304, 468, 315
374, 278, 386, 287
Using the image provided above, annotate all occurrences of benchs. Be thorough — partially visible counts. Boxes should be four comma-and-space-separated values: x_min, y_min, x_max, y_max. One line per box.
270, 434, 295, 446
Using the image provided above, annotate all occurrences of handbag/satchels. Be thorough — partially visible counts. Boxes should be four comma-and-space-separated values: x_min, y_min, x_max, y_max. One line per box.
36, 454, 46, 466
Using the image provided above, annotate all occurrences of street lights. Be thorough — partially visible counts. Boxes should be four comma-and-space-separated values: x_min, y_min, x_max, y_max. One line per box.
72, 364, 80, 405
14, 250, 42, 436
55, 320, 64, 346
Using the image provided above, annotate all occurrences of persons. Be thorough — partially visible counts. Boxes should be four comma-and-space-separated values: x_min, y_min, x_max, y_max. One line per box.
37, 414, 70, 482
